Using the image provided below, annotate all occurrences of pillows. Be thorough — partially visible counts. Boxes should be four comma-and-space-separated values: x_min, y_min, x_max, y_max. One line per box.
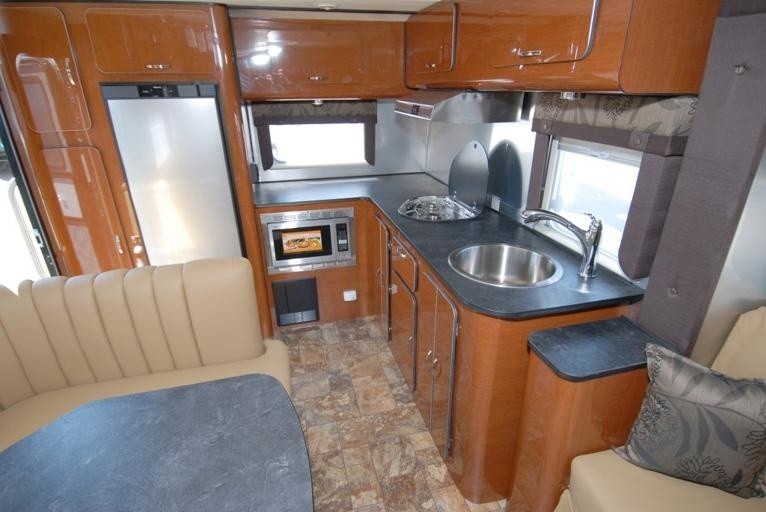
610, 341, 765, 497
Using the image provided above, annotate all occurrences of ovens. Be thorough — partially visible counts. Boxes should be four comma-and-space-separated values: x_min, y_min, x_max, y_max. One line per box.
258, 207, 357, 276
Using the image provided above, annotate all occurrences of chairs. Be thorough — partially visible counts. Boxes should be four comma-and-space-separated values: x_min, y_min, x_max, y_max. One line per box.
553, 304, 765, 512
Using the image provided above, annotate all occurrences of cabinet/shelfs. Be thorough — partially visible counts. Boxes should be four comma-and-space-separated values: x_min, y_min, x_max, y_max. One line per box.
368, 199, 391, 347
83, 8, 218, 75
231, 17, 407, 99
405, 1, 720, 96
390, 221, 421, 403
417, 256, 640, 503
0, 0, 135, 275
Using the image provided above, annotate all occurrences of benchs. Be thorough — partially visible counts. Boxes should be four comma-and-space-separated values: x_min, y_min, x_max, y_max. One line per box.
1, 257, 292, 451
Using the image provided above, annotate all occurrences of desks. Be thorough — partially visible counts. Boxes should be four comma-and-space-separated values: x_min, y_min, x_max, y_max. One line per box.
1, 372, 314, 512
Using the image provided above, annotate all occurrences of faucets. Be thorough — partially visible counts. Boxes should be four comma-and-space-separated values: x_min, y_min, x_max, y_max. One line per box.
519, 208, 604, 278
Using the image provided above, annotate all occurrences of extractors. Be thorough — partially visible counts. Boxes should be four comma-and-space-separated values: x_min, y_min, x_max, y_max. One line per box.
390, 88, 525, 126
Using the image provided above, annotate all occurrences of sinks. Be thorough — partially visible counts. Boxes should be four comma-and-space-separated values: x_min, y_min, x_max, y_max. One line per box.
447, 240, 563, 289
